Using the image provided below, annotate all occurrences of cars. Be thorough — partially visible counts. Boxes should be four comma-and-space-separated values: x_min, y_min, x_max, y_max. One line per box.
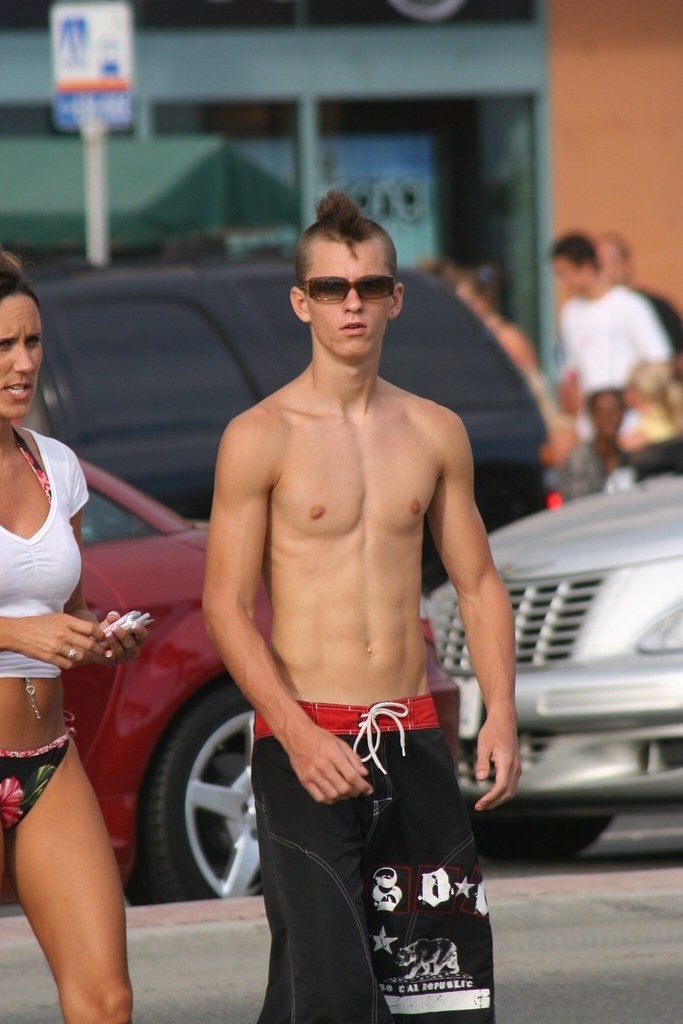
38, 434, 460, 907
427, 469, 683, 861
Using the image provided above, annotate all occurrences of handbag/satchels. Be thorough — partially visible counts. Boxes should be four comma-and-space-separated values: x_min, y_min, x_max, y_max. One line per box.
615, 362, 683, 453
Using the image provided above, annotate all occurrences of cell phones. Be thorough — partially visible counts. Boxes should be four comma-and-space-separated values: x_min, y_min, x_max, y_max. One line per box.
89, 608, 155, 651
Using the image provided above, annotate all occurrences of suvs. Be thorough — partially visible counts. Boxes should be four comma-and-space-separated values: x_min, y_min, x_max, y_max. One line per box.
31, 255, 570, 595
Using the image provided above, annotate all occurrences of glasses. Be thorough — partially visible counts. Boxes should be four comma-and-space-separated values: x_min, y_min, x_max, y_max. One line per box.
299, 274, 395, 301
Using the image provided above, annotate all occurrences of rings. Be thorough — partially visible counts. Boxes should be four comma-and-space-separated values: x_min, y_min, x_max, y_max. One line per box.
67, 648, 76, 659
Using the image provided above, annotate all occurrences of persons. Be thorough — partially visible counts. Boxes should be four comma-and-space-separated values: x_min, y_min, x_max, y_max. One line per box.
416, 227, 683, 504
1, 248, 151, 1024
202, 192, 522, 1024
